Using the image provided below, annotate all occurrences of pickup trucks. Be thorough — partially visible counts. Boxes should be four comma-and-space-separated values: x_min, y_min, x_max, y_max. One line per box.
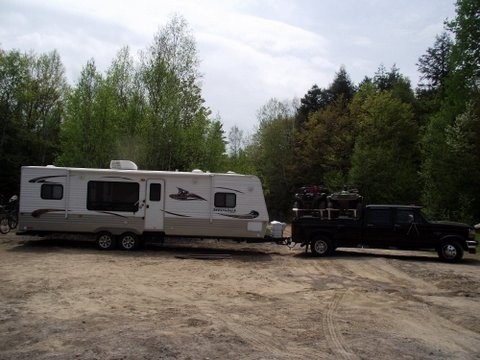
290, 199, 479, 262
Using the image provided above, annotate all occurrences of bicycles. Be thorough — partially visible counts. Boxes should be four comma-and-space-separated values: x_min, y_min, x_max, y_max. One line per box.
0, 200, 19, 234
